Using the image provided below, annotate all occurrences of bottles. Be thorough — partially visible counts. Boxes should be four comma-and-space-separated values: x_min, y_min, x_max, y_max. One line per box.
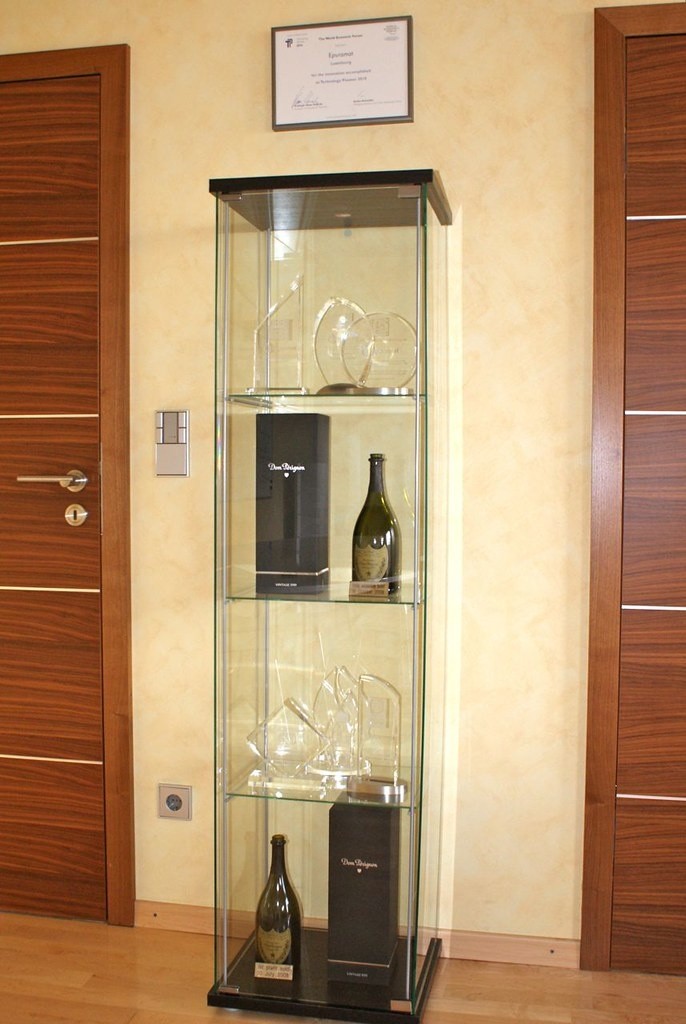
353, 453, 401, 594
255, 834, 301, 968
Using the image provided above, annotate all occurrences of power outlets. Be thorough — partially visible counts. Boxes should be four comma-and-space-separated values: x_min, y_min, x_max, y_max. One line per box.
158, 784, 192, 820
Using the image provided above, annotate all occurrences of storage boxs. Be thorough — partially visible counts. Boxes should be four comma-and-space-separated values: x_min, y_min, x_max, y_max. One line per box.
257, 413, 331, 594
328, 791, 401, 986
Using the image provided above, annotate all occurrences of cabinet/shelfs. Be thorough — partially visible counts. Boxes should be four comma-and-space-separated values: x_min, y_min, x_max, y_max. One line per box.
205, 169, 452, 1024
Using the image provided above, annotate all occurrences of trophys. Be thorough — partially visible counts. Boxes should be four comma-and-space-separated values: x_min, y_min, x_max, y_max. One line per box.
245, 666, 405, 796
245, 274, 417, 395
